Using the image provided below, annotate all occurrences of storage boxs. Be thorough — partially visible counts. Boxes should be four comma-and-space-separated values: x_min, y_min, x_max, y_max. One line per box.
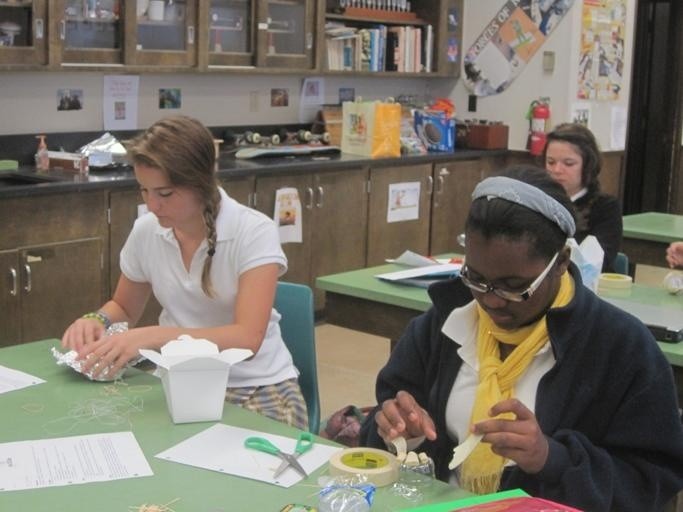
465, 123, 510, 151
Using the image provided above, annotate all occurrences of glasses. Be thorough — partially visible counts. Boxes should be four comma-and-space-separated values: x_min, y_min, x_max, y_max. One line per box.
459, 251, 564, 302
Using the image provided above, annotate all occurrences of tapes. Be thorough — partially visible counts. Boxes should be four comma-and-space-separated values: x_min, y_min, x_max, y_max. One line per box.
329, 435, 407, 487
600, 273, 632, 289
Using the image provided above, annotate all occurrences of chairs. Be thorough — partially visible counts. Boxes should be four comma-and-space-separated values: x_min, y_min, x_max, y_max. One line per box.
269, 281, 321, 437
633, 263, 683, 290
604, 250, 629, 278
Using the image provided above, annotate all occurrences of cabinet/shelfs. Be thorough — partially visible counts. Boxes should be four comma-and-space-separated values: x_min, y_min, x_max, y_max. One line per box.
44, 1, 123, 74
121, 4, 197, 79
0, 2, 47, 72
366, 154, 507, 270
0, 185, 111, 349
195, 2, 254, 79
256, 1, 317, 79
322, 3, 463, 79
252, 163, 368, 327
106, 175, 257, 329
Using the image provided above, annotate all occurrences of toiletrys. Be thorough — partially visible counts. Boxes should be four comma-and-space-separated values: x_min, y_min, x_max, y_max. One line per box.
33, 135, 50, 171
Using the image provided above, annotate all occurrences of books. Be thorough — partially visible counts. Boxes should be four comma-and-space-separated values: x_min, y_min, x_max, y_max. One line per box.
374, 264, 465, 289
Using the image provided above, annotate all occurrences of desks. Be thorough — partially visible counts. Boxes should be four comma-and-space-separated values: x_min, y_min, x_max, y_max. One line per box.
0, 337, 484, 512
618, 210, 683, 275
311, 248, 683, 419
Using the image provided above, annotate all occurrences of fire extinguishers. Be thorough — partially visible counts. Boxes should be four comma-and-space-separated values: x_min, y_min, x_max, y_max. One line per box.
526, 96, 551, 156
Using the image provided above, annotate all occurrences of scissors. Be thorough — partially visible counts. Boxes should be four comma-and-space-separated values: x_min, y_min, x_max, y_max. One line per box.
244, 431, 314, 478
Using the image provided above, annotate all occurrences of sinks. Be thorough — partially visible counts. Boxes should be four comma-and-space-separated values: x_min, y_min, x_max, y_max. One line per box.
0, 169, 56, 188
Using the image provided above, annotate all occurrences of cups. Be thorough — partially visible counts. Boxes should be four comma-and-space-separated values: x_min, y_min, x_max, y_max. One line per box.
396, 457, 438, 501
135, 0, 185, 23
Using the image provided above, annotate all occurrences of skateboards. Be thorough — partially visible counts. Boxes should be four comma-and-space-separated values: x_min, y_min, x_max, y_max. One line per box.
220, 128, 341, 159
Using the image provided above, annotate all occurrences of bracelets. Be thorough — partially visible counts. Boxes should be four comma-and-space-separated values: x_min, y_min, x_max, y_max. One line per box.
81, 312, 111, 329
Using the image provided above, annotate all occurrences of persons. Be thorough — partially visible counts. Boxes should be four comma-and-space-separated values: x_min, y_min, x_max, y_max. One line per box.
60, 113, 308, 437
326, 22, 435, 73
540, 121, 623, 273
362, 164, 683, 512
